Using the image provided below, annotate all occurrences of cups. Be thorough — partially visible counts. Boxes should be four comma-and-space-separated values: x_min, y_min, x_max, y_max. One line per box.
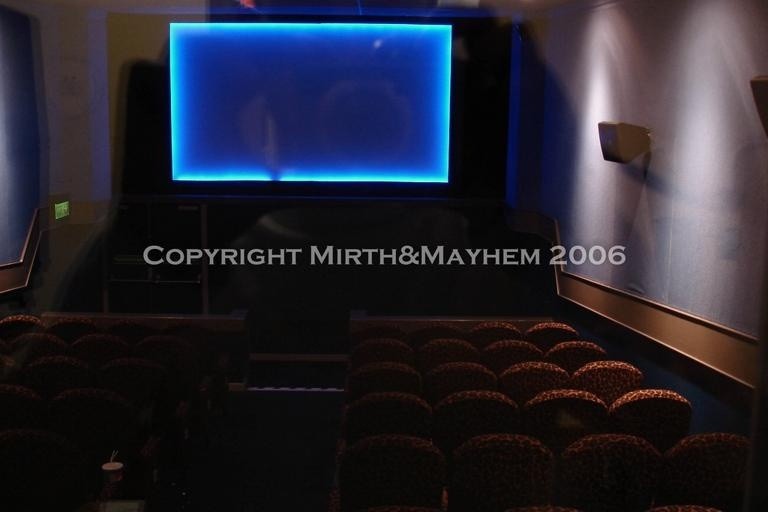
101, 462, 124, 482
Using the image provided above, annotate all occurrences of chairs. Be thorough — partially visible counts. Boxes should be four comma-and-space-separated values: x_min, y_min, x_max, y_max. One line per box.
327, 316, 754, 512
0, 312, 236, 512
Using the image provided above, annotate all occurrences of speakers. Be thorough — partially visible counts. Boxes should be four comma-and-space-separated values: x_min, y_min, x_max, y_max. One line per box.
750, 75, 768, 136
598, 121, 650, 164
